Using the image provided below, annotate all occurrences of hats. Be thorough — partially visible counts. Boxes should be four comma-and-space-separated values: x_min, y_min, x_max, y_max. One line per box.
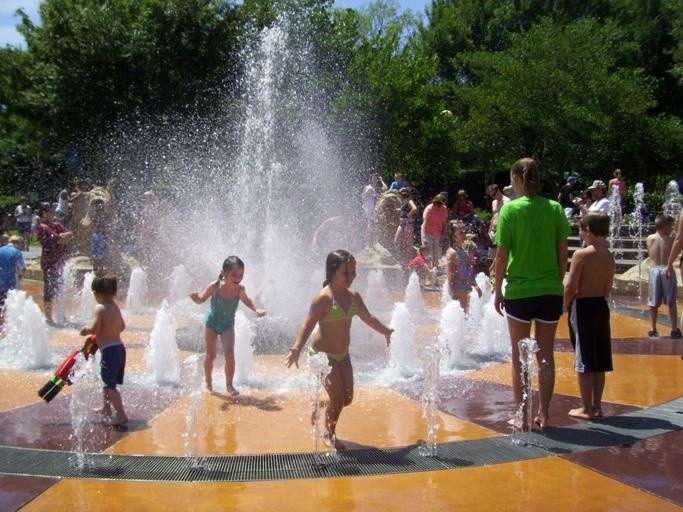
567, 177, 576, 182
588, 180, 606, 190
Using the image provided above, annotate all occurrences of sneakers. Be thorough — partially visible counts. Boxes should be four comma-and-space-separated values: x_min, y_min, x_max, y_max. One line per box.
648, 331, 658, 337
671, 328, 682, 339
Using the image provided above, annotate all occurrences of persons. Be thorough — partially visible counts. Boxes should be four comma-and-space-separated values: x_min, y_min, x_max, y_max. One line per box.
0, 155, 683, 451
